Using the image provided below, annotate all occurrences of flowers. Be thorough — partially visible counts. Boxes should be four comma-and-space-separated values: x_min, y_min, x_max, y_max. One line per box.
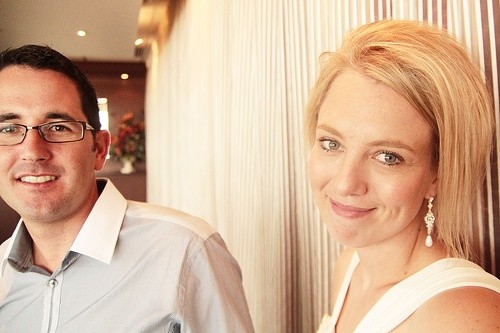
108, 111, 145, 161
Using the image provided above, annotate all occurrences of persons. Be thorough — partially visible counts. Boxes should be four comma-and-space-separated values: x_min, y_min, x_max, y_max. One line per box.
0, 44, 256, 333
307, 18, 500, 333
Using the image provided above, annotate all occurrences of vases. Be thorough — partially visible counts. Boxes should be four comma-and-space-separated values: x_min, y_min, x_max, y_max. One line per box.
120, 161, 138, 174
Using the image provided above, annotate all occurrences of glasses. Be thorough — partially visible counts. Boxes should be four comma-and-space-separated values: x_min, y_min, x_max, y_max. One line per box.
1, 120, 94, 146
